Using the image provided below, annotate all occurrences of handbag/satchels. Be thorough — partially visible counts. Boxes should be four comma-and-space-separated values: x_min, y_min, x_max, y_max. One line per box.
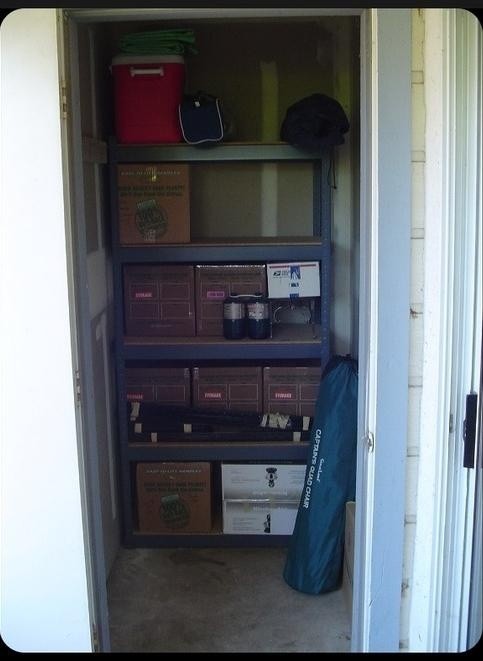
176, 88, 229, 146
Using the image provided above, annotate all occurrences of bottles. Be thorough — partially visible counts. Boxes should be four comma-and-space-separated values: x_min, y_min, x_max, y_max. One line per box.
222, 291, 270, 342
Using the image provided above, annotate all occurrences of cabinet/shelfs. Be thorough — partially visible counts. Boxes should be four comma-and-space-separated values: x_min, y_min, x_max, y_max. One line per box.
91, 16, 333, 554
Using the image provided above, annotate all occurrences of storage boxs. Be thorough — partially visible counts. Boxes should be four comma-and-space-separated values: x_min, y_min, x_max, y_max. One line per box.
133, 461, 306, 537
124, 365, 319, 421
115, 163, 192, 246
124, 262, 267, 339
110, 52, 186, 144
